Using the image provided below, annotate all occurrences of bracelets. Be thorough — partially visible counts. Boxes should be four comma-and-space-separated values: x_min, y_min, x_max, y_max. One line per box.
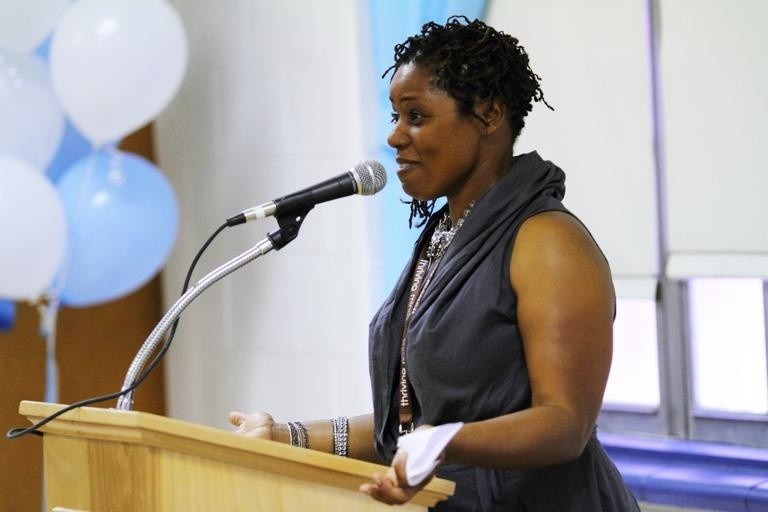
332, 416, 348, 457
285, 421, 309, 448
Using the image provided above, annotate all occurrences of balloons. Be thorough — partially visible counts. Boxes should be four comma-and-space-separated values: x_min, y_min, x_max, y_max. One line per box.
0, 0, 190, 331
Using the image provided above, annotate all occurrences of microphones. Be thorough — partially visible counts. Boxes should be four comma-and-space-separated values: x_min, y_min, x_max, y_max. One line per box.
227, 160, 387, 227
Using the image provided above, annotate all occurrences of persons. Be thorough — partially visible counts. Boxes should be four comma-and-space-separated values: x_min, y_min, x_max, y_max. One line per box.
227, 15, 642, 512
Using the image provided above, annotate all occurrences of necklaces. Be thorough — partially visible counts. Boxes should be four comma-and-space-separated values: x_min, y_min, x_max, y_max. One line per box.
426, 201, 476, 267
